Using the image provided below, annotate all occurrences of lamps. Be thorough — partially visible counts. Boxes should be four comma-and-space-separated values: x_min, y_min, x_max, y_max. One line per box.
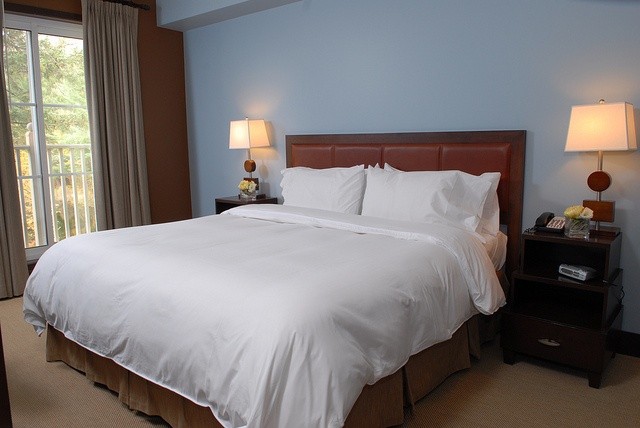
563, 99, 639, 238
228, 116, 272, 199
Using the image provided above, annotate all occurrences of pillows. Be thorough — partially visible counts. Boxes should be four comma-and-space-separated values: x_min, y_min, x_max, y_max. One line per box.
361, 164, 491, 230
280, 164, 364, 216
384, 163, 501, 236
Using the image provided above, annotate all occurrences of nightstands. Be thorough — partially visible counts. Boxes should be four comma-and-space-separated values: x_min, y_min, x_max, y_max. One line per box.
500, 223, 624, 388
215, 195, 278, 213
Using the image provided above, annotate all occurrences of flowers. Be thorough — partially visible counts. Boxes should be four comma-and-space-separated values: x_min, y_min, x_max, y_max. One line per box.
564, 205, 594, 231
238, 180, 256, 195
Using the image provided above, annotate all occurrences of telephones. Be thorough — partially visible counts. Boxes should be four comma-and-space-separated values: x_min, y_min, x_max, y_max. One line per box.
525, 212, 565, 233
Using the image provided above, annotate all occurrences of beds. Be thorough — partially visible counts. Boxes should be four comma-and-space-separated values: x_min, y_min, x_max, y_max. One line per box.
22, 130, 529, 428
